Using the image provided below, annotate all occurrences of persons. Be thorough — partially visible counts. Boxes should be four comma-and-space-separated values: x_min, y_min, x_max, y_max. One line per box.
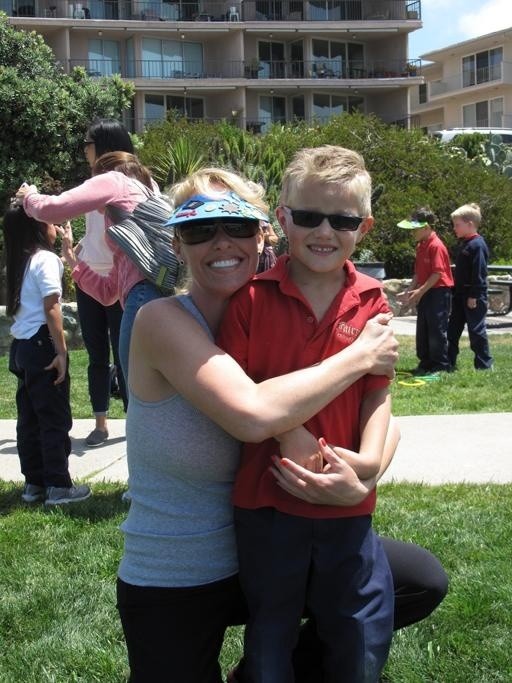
5, 205, 90, 503
115, 151, 451, 680
256, 223, 278, 274
447, 198, 498, 378
9, 118, 174, 447
214, 141, 398, 683
393, 202, 455, 383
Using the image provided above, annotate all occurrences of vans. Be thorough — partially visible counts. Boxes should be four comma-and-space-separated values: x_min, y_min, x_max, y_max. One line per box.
429, 125, 512, 150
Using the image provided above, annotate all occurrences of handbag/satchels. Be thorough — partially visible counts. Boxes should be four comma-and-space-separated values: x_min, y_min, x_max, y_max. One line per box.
106, 179, 180, 293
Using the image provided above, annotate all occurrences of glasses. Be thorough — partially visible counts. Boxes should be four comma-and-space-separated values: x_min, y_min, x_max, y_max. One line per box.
176, 219, 260, 245
284, 208, 364, 232
81, 138, 95, 150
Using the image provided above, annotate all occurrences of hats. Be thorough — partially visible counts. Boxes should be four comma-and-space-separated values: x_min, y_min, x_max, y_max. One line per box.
396, 218, 427, 230
161, 184, 270, 228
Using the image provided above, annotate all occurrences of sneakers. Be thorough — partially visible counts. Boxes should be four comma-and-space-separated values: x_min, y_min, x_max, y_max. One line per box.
416, 367, 442, 376
85, 427, 109, 447
43, 483, 93, 504
20, 480, 48, 503
